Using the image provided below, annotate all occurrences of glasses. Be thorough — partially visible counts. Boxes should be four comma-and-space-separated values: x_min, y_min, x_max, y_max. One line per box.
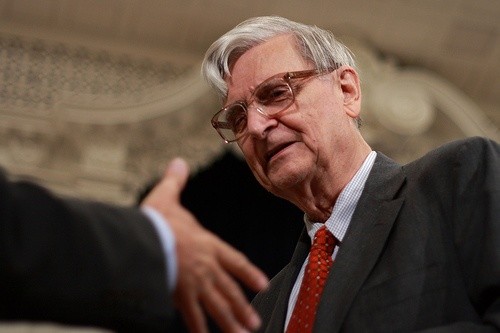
210, 66, 339, 143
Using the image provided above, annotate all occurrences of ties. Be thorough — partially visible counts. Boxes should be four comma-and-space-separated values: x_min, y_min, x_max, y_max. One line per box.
284, 226, 339, 333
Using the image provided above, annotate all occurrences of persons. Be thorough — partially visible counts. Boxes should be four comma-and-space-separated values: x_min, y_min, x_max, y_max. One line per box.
0, 156, 270, 333
200, 17, 500, 333
